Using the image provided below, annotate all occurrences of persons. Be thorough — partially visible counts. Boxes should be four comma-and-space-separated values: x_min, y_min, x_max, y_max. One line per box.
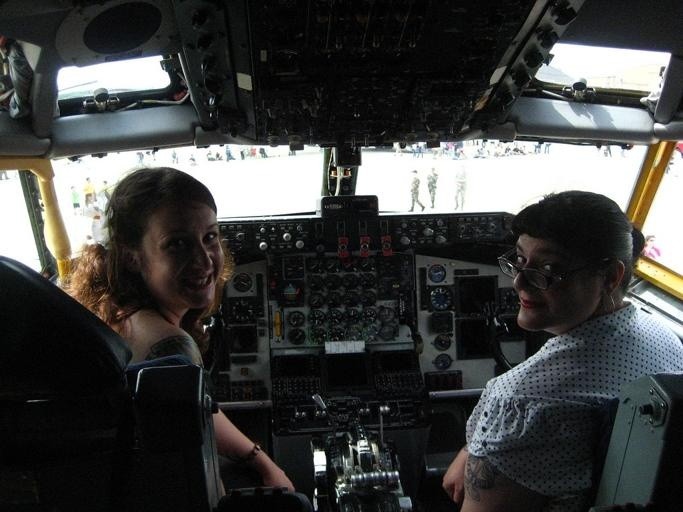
453, 179, 463, 210
68, 138, 296, 242
427, 167, 438, 208
637, 232, 663, 262
73, 163, 295, 496
407, 170, 426, 211
411, 140, 551, 160
439, 190, 683, 512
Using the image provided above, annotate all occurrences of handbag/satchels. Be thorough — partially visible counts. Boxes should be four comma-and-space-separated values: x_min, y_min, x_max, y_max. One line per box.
240, 442, 262, 468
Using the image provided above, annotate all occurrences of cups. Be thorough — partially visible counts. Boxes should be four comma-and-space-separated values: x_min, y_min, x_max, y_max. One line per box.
497, 244, 612, 291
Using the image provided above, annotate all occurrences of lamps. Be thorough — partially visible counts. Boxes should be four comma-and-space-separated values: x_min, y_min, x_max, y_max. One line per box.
590, 371, 683, 509
0, 260, 226, 509
